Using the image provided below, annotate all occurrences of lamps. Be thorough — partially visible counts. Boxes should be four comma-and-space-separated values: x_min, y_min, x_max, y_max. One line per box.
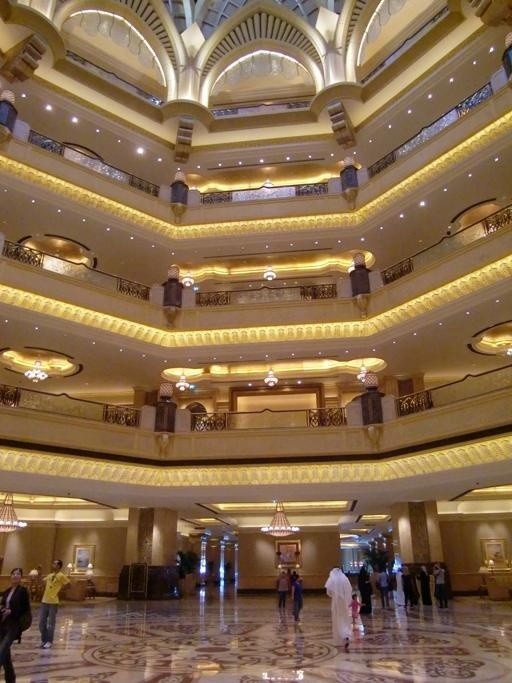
263, 177, 273, 187
264, 369, 278, 387
261, 499, 299, 537
175, 373, 189, 392
181, 271, 194, 287
24, 358, 48, 383
2, 492, 27, 532
349, 260, 354, 272
263, 265, 276, 281
356, 365, 368, 383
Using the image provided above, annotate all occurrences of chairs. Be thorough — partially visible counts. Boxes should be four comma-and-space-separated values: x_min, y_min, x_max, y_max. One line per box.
25, 572, 96, 601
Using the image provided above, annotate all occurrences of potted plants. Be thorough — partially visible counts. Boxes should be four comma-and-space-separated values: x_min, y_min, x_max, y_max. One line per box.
175, 549, 232, 596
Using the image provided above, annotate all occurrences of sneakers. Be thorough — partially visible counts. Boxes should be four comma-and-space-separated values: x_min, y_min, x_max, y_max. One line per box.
43, 640, 52, 647
35, 641, 45, 647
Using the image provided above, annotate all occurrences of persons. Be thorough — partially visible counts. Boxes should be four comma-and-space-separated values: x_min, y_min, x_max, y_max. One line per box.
348, 594, 365, 622
291, 576, 304, 621
37, 560, 74, 649
394, 565, 448, 609
326, 566, 354, 652
276, 571, 288, 609
289, 570, 300, 600
0, 568, 32, 683
358, 563, 372, 612
376, 567, 392, 607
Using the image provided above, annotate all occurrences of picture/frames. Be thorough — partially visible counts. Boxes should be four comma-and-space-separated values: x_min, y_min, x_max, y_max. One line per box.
479, 538, 507, 565
72, 544, 96, 574
276, 539, 301, 568
